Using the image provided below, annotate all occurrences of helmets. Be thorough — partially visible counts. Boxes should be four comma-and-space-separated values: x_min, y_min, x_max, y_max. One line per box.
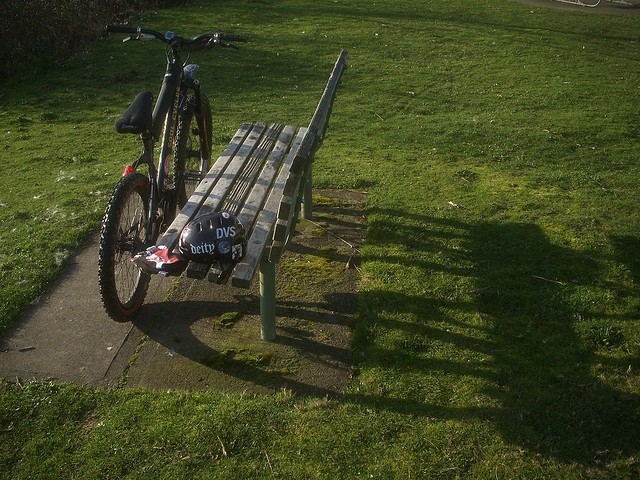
178, 211, 247, 263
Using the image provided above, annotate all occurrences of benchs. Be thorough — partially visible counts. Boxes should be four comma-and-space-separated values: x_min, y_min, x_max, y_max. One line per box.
135, 48, 349, 341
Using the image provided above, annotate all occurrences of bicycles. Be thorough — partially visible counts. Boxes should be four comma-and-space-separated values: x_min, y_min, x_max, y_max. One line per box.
99, 24, 248, 322
